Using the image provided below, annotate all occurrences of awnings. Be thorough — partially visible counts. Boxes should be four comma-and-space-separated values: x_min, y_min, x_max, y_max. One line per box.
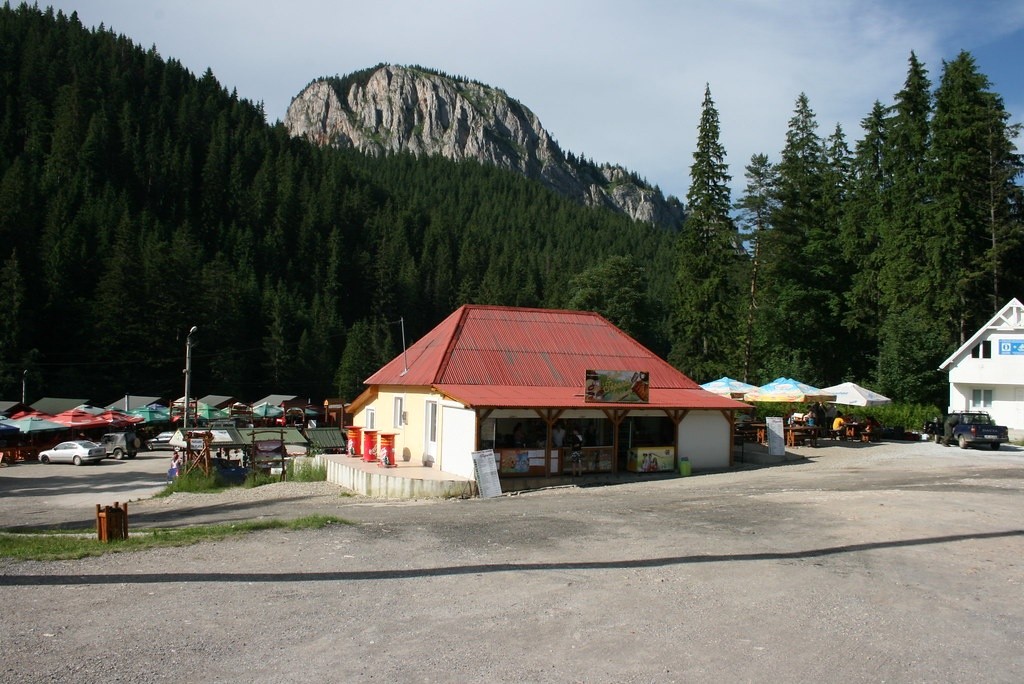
430, 383, 758, 477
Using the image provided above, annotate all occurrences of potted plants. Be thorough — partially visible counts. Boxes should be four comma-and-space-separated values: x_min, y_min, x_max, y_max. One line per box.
97, 502, 127, 543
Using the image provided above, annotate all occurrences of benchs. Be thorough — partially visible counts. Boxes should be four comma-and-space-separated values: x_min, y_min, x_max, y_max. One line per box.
860, 431, 880, 442
829, 430, 845, 442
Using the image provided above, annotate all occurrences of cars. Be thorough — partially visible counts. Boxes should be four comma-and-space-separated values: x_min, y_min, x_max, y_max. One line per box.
38, 439, 108, 466
144, 432, 186, 452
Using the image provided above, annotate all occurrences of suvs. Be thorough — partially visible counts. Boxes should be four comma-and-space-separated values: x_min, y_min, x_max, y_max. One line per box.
94, 432, 141, 460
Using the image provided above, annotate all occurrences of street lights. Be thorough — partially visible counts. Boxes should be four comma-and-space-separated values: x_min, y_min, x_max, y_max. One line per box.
183, 325, 197, 428
22, 369, 29, 403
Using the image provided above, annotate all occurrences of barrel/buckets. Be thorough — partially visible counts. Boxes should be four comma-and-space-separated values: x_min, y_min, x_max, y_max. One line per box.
679, 455, 691, 476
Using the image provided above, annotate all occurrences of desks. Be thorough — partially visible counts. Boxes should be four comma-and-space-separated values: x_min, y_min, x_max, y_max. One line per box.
841, 422, 865, 442
733, 421, 767, 443
783, 426, 825, 447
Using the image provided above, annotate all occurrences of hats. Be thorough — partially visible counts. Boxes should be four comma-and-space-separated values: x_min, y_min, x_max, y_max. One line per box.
837, 411, 843, 417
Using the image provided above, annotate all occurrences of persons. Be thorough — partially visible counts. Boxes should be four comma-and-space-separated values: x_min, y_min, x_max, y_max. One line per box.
641, 453, 658, 471
170, 454, 181, 476
513, 422, 525, 448
803, 402, 850, 446
607, 423, 613, 446
942, 415, 959, 446
584, 422, 597, 446
859, 416, 881, 441
568, 428, 583, 477
551, 421, 567, 447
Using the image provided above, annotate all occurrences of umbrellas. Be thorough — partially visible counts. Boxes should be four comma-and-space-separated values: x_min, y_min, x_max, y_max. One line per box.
698, 377, 891, 417
0, 397, 317, 448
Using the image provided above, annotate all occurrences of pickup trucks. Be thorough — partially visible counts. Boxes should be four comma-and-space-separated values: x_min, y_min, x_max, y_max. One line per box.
923, 411, 1009, 449
